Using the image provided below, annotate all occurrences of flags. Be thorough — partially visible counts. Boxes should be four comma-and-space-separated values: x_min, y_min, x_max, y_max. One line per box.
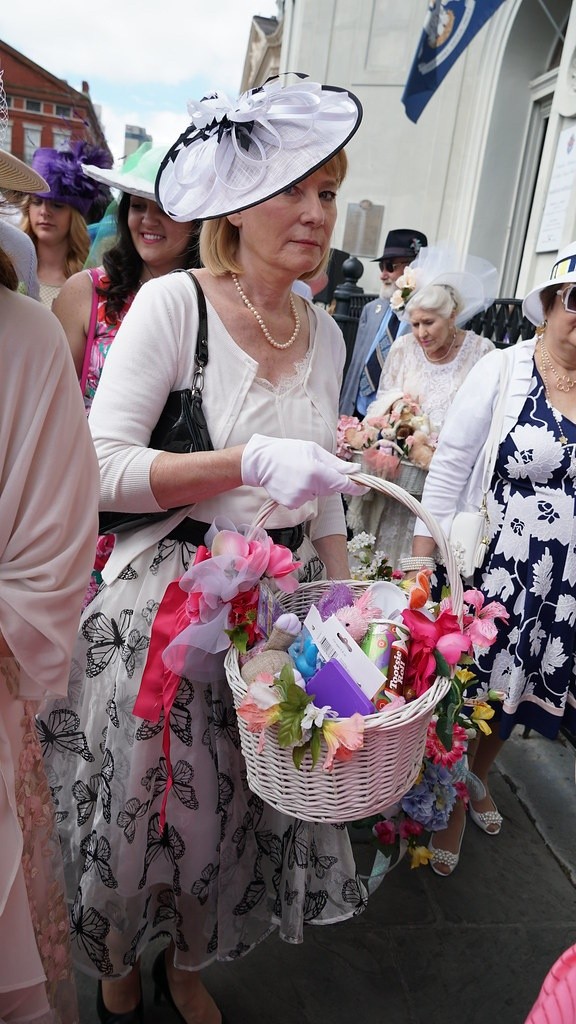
401, 0, 509, 124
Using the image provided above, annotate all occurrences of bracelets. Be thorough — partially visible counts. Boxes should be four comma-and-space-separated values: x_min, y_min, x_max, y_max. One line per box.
398, 557, 437, 572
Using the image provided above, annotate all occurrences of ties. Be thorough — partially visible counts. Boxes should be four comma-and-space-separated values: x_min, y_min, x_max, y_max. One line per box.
360, 308, 402, 398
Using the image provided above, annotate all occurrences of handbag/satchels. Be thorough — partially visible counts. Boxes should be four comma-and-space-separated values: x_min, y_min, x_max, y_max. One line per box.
433, 350, 511, 579
96, 270, 214, 537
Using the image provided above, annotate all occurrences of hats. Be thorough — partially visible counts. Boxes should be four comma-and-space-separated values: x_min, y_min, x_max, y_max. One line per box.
28, 139, 111, 217
0, 148, 50, 195
522, 242, 576, 327
372, 229, 429, 260
80, 146, 164, 200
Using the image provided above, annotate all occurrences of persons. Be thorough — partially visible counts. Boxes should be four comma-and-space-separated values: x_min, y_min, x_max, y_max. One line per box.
0, 148, 100, 1024
38, 71, 371, 1024
0, 106, 110, 307
51, 138, 205, 614
346, 246, 501, 573
339, 229, 428, 421
411, 238, 576, 876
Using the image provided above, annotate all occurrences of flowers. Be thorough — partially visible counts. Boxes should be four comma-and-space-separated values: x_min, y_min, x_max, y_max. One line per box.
233, 665, 364, 772
161, 517, 305, 681
389, 265, 421, 312
336, 400, 437, 477
404, 568, 512, 868
349, 532, 403, 587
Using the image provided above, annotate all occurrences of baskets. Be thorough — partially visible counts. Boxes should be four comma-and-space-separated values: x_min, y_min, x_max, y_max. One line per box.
349, 403, 430, 494
223, 469, 462, 822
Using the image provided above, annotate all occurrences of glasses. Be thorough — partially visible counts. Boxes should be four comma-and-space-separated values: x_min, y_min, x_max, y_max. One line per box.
557, 285, 576, 313
378, 260, 407, 272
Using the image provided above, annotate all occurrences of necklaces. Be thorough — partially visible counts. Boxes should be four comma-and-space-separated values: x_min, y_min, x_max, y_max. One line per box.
138, 277, 142, 284
540, 329, 576, 468
424, 326, 458, 362
231, 272, 301, 350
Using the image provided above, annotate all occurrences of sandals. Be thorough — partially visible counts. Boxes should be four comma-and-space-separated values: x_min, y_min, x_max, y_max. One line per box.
470, 793, 501, 834
428, 813, 467, 875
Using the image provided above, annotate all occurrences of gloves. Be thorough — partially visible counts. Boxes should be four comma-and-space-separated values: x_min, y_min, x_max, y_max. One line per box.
242, 433, 369, 509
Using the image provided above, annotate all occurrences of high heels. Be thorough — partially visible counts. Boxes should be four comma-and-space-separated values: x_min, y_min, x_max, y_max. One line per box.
95, 958, 146, 1024
152, 954, 225, 1024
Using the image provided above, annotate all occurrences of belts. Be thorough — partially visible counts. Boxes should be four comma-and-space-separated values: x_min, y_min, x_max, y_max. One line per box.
171, 517, 304, 550
